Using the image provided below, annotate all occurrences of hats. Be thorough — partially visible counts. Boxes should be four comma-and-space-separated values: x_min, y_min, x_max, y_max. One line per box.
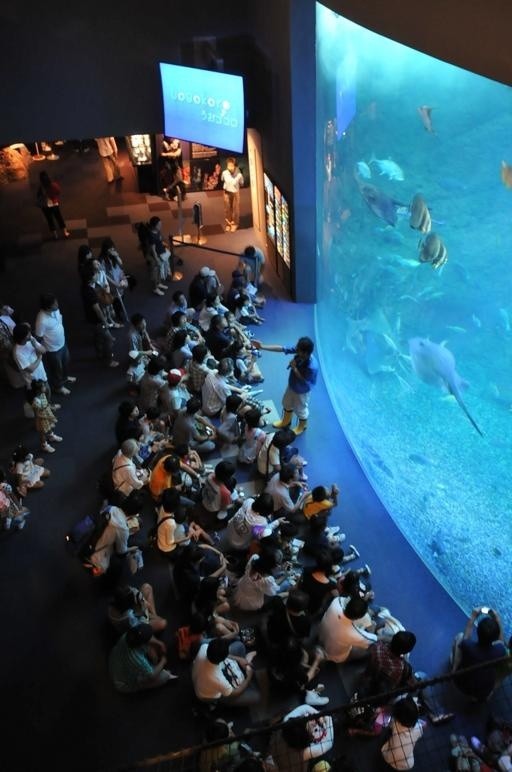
209, 270, 215, 276
168, 367, 185, 384
128, 351, 143, 362
200, 266, 209, 276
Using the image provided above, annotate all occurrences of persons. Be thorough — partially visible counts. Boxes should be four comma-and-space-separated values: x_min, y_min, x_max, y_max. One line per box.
276, 634, 331, 707
250, 336, 320, 436
98, 238, 129, 327
188, 343, 218, 396
105, 557, 167, 637
262, 463, 311, 519
11, 441, 51, 490
221, 157, 244, 233
139, 355, 169, 412
172, 396, 218, 452
225, 491, 290, 553
140, 406, 167, 436
235, 551, 288, 616
237, 407, 268, 465
449, 710, 512, 772
302, 483, 340, 522
267, 588, 312, 657
217, 394, 244, 445
125, 349, 147, 387
173, 504, 221, 549
173, 542, 230, 605
107, 623, 181, 692
258, 535, 285, 567
448, 605, 510, 707
230, 476, 244, 508
149, 453, 207, 509
156, 486, 187, 569
268, 703, 335, 772
198, 292, 231, 333
329, 543, 372, 582
0, 302, 21, 373
205, 313, 235, 357
196, 461, 237, 531
302, 547, 338, 620
267, 454, 309, 503
159, 367, 194, 415
159, 133, 187, 203
377, 697, 426, 771
188, 267, 225, 309
305, 511, 346, 558
144, 215, 169, 297
12, 321, 62, 411
226, 245, 266, 326
88, 257, 125, 329
168, 329, 194, 369
35, 168, 72, 243
166, 289, 196, 324
257, 427, 294, 480
112, 438, 153, 497
194, 575, 232, 614
365, 630, 417, 692
82, 494, 145, 601
279, 523, 300, 564
94, 136, 125, 185
201, 356, 252, 420
198, 716, 281, 772
188, 611, 256, 647
342, 690, 456, 739
34, 292, 77, 396
78, 244, 94, 279
115, 400, 150, 442
165, 311, 207, 351
125, 313, 166, 374
315, 595, 391, 671
336, 570, 373, 597
0, 471, 27, 531
31, 380, 64, 454
82, 258, 121, 370
190, 638, 263, 709
207, 156, 224, 191
222, 338, 262, 384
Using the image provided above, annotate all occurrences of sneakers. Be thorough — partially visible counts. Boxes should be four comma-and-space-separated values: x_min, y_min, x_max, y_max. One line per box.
158, 283, 168, 290
51, 404, 61, 409
305, 690, 329, 706
109, 361, 119, 368
365, 564, 371, 576
50, 435, 63, 442
349, 544, 360, 560
315, 683, 325, 691
337, 534, 346, 542
41, 444, 56, 453
330, 526, 339, 533
431, 712, 455, 725
66, 376, 76, 383
54, 387, 71, 394
153, 288, 164, 296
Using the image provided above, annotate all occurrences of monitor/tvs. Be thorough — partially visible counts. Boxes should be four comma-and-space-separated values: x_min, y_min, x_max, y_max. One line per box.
158, 60, 243, 156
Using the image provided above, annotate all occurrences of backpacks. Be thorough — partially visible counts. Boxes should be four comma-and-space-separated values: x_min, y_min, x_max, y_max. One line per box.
177, 623, 205, 662
64, 504, 114, 560
146, 515, 177, 556
96, 465, 131, 499
238, 426, 262, 464
23, 399, 36, 420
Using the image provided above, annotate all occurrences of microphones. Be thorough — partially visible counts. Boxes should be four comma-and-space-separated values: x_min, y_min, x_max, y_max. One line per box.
286, 355, 299, 369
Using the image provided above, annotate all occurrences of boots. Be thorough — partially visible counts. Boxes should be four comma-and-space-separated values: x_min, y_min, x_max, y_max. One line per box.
292, 419, 307, 435
271, 409, 292, 428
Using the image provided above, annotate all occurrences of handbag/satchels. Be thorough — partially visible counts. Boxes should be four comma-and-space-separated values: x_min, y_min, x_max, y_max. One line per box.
92, 279, 115, 307
